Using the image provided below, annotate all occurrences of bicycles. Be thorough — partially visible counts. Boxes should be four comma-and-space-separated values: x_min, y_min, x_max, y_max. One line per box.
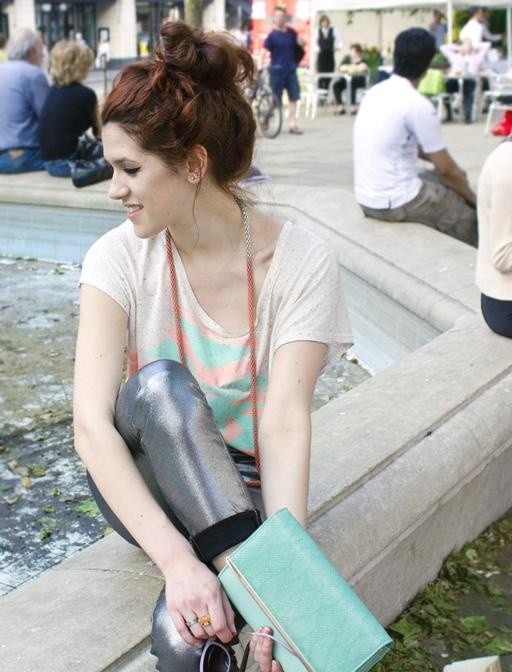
238, 64, 290, 143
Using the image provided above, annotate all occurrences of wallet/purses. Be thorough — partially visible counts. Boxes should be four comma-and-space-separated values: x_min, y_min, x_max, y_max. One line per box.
215, 507, 394, 672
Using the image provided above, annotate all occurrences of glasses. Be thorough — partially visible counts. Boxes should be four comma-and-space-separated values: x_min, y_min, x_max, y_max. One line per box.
199, 630, 297, 672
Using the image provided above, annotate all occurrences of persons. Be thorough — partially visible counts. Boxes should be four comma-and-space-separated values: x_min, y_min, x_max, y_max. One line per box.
36, 38, 103, 178
233, 7, 504, 136
0, 27, 50, 175
350, 27, 479, 252
71, 21, 355, 672
474, 107, 511, 339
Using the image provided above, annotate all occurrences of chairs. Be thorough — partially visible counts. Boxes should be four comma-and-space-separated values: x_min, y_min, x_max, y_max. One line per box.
283, 50, 512, 135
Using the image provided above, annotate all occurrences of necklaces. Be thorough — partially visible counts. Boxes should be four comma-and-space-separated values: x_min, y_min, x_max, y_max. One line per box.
166, 195, 261, 489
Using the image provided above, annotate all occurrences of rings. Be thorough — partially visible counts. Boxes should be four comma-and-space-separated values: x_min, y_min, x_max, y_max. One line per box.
186, 618, 198, 628
198, 615, 211, 626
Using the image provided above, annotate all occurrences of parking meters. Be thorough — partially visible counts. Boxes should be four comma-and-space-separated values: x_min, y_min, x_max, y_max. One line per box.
97, 42, 111, 98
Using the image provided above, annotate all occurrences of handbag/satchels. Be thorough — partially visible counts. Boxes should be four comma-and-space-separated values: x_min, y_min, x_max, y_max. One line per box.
71, 150, 113, 187
